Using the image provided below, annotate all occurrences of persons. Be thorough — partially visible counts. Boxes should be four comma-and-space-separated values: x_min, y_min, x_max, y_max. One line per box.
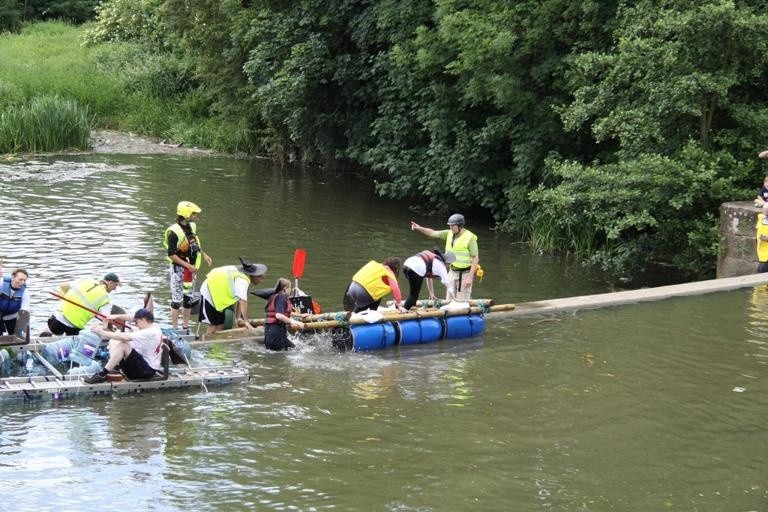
755, 203, 768, 273
266, 276, 304, 351
403, 247, 455, 309
163, 199, 212, 332
343, 257, 405, 314
754, 176, 768, 207
410, 211, 481, 304
0, 264, 31, 338
82, 307, 165, 384
197, 260, 266, 336
42, 273, 121, 336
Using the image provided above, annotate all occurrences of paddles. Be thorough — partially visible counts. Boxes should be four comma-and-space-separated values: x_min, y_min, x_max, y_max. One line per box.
293, 249, 306, 297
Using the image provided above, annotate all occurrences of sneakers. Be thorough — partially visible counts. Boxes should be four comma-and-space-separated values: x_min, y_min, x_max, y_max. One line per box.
84, 372, 106, 384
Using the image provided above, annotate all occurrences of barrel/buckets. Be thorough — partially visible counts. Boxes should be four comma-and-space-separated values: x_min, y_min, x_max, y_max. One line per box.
442, 315, 486, 339
42, 338, 76, 366
69, 326, 103, 366
393, 320, 444, 345
345, 321, 395, 352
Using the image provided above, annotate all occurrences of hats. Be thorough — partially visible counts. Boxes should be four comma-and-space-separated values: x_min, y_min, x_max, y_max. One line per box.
104, 272, 121, 287
132, 307, 151, 323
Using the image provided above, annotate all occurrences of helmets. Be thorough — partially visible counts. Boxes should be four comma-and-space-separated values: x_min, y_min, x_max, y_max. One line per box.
446, 213, 466, 228
177, 200, 202, 221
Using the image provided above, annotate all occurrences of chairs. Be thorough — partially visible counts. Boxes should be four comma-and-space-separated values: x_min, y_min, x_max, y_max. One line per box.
120, 344, 171, 382
102, 305, 127, 341
0, 310, 30, 346
